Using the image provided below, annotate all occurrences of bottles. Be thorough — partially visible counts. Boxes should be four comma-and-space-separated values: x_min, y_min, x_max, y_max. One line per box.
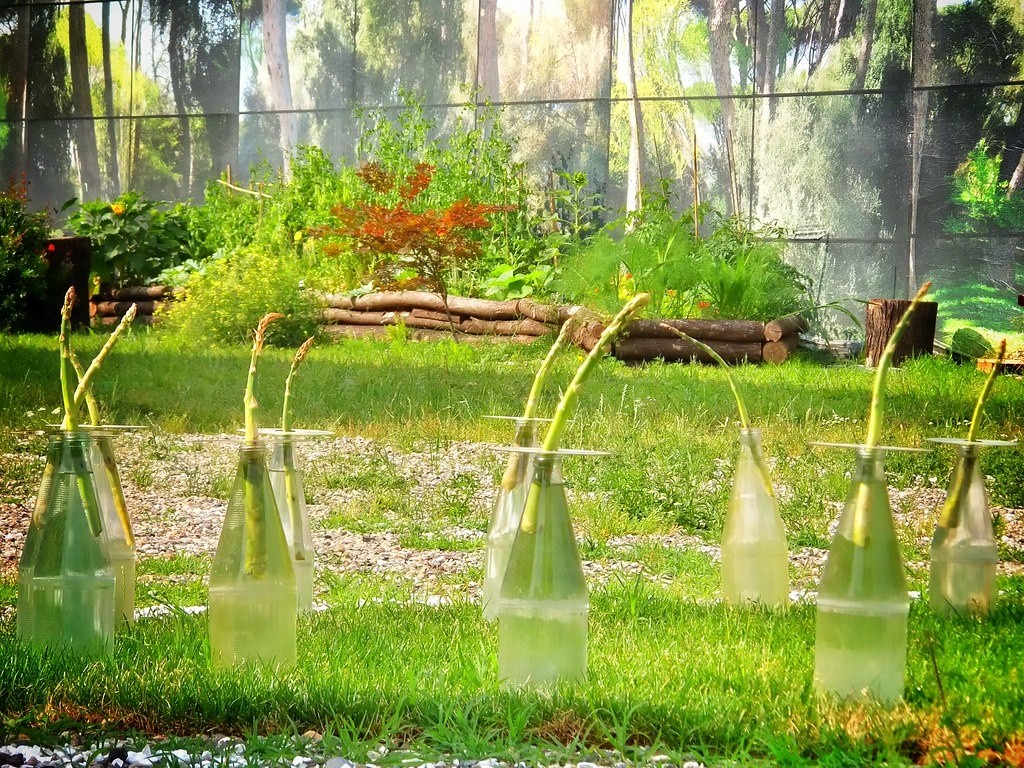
929, 444, 997, 616
208, 441, 297, 671
497, 456, 587, 689
271, 435, 312, 636
814, 447, 910, 704
722, 429, 789, 608
482, 420, 535, 621
90, 432, 135, 626
17, 431, 115, 659
